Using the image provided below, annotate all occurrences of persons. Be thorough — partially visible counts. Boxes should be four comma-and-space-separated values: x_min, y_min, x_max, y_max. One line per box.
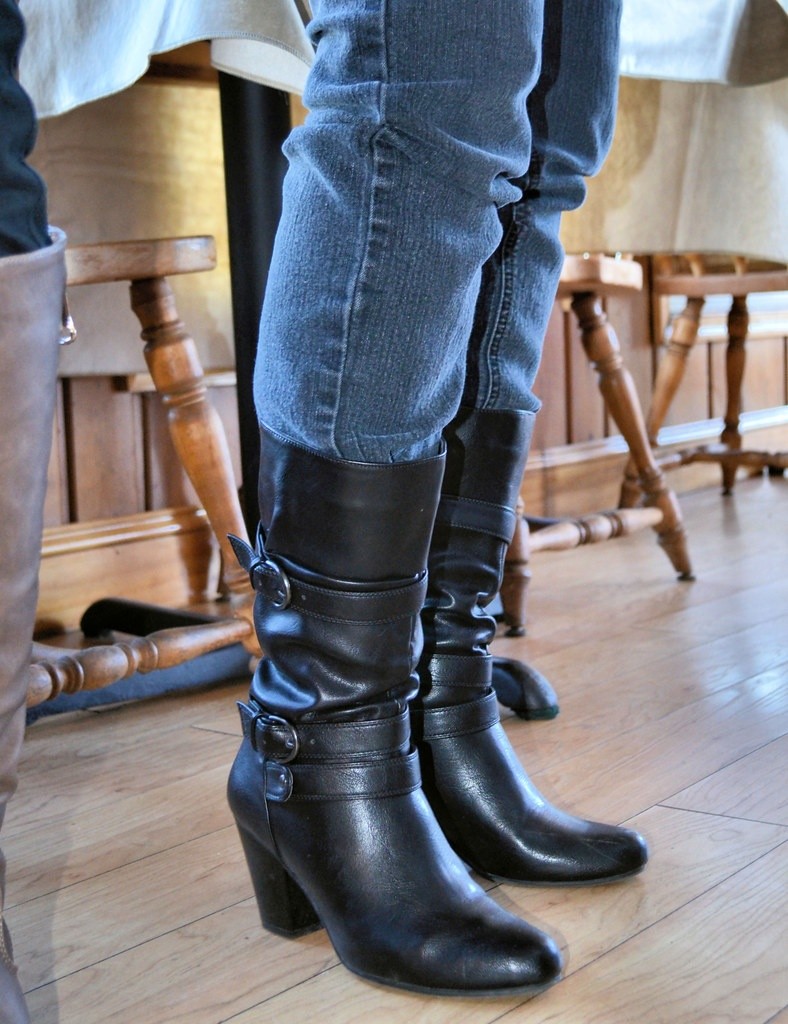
0, 0, 68, 1023
224, 0, 649, 998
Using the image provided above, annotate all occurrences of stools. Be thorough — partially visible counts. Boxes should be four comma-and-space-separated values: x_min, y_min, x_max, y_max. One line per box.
26, 236, 265, 708
498, 255, 696, 637
621, 249, 787, 506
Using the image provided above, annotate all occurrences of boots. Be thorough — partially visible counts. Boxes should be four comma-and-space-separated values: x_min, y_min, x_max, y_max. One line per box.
0, 226, 68, 1023
409, 410, 648, 883
226, 423, 563, 995
